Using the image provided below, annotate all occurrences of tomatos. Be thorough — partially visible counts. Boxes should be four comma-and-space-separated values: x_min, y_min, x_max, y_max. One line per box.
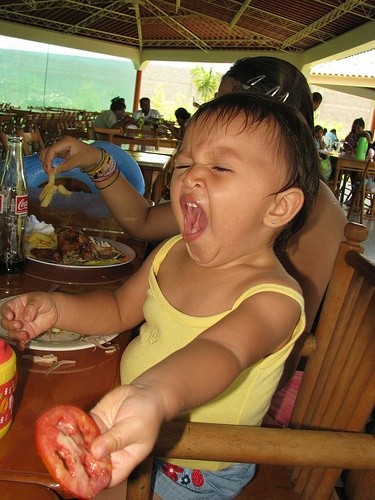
35, 405, 112, 499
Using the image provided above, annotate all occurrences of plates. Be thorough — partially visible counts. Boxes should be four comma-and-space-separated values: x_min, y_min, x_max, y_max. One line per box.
0, 296, 119, 352
26, 235, 135, 269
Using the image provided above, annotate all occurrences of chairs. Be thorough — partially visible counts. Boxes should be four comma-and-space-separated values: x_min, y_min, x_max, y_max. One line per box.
0, 107, 375, 500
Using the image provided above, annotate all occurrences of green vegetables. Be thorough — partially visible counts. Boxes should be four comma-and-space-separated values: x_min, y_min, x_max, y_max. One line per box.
63, 252, 121, 265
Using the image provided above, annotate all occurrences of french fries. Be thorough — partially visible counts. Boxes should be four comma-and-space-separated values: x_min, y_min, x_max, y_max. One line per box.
23, 230, 57, 254
40, 170, 72, 207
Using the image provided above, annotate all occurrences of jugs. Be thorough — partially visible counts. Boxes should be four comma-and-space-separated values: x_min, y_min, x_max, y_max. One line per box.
355, 138, 367, 161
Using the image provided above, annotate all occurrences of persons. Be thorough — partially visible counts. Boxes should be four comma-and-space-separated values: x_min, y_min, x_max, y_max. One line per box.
1, 94, 319, 500
193, 92, 218, 109
311, 92, 339, 162
92, 96, 161, 141
343, 117, 375, 211
36, 56, 346, 390
162, 107, 191, 140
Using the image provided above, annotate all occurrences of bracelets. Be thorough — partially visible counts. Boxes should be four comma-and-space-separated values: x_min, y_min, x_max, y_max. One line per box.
81, 147, 122, 187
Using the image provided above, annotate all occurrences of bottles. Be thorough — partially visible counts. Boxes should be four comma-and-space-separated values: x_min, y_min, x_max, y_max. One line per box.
0, 339, 17, 438
138, 116, 144, 130
0, 136, 27, 276
159, 115, 164, 129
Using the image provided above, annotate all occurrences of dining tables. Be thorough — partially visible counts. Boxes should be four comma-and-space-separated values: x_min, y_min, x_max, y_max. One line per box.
81, 139, 178, 187
124, 150, 171, 206
0, 112, 17, 135
319, 148, 375, 205
46, 109, 82, 129
12, 108, 60, 131
0, 183, 150, 500
